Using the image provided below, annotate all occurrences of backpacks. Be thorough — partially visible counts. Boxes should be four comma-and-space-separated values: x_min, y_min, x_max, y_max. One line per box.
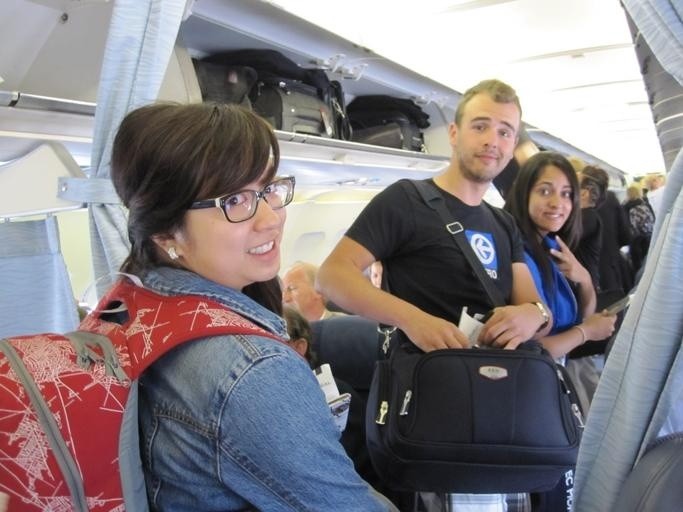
0, 273, 294, 512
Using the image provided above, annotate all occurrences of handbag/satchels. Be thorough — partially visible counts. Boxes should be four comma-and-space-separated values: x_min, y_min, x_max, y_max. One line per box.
303, 65, 353, 142
344, 92, 431, 130
202, 46, 326, 88
363, 323, 585, 494
192, 52, 260, 105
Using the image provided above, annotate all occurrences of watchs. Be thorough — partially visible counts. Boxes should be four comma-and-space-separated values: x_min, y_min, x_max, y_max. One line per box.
531, 296, 550, 331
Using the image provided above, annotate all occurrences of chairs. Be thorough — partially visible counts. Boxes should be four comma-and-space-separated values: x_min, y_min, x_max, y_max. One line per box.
608, 430, 683, 512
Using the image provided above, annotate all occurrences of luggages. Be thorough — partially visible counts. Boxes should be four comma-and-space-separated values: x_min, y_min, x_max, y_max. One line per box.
351, 106, 425, 153
250, 73, 340, 143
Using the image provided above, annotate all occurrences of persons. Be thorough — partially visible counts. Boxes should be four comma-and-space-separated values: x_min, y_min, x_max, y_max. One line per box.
571, 167, 666, 420
511, 153, 620, 393
276, 309, 374, 478
280, 259, 349, 324
311, 79, 552, 509
367, 257, 384, 291
103, 82, 395, 511
491, 146, 600, 412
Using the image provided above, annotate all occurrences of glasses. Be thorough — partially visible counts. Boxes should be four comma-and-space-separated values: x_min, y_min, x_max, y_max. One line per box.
183, 174, 296, 223
